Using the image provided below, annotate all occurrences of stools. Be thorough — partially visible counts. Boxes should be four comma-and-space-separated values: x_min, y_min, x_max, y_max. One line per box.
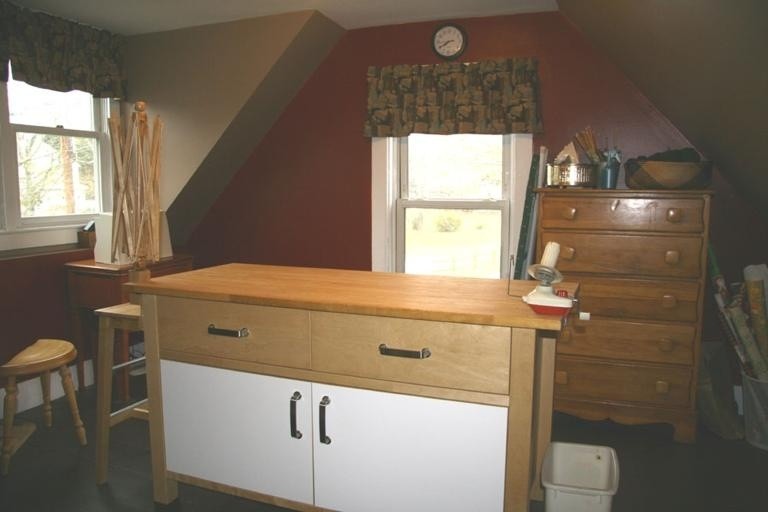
1, 302, 149, 487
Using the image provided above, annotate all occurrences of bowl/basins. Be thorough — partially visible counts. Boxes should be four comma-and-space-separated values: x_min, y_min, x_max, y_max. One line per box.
625, 160, 711, 191
546, 162, 598, 188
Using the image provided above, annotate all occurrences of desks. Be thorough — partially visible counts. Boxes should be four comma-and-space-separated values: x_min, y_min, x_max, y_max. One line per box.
63, 248, 198, 404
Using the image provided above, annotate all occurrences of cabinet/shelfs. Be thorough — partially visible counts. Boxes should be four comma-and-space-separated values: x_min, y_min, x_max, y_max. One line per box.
122, 263, 580, 512
534, 187, 711, 442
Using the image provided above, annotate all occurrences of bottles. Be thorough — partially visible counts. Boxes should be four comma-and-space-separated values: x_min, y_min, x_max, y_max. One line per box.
602, 153, 616, 188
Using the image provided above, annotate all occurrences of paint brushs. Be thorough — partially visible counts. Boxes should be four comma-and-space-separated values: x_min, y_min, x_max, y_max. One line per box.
575, 128, 600, 164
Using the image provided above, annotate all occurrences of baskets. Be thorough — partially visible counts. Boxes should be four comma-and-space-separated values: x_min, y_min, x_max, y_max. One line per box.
621, 157, 714, 191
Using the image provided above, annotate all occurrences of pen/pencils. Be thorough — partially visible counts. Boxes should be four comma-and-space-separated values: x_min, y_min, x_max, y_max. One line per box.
600, 145, 622, 165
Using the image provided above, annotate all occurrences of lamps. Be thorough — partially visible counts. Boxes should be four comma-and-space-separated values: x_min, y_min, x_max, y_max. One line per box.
521, 242, 577, 315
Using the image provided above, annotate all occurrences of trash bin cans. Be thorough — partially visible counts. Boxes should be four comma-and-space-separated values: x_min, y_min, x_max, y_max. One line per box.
541, 441, 621, 512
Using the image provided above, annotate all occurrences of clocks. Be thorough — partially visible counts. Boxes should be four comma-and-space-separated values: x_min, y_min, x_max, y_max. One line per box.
430, 22, 469, 61
